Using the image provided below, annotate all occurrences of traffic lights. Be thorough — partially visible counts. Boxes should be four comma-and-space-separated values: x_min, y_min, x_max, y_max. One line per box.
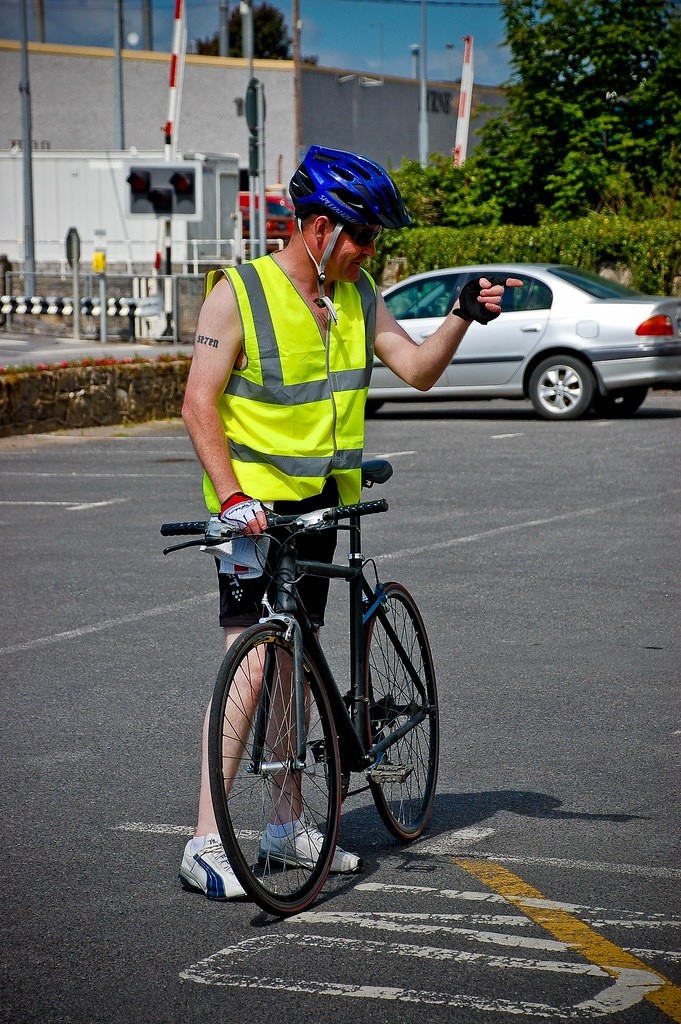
126, 165, 198, 215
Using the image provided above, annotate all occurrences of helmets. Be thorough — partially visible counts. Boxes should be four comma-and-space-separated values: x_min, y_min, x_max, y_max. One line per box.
290, 144, 411, 228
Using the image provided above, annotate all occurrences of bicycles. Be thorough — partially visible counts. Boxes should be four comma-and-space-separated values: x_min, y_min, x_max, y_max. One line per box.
158, 456, 441, 916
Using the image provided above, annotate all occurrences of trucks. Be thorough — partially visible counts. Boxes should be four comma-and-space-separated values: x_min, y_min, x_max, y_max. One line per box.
0, 144, 237, 275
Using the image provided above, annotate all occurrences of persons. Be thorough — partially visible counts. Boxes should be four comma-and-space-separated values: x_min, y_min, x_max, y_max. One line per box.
176, 145, 526, 902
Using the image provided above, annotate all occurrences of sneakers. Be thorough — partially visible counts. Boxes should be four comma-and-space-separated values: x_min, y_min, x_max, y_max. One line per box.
178, 833, 264, 900
258, 813, 363, 874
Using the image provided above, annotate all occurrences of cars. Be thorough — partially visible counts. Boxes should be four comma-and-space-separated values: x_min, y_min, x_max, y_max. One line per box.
366, 263, 680, 419
239, 190, 298, 242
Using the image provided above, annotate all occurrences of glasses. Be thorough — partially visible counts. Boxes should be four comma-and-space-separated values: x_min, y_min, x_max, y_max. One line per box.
332, 213, 382, 248
